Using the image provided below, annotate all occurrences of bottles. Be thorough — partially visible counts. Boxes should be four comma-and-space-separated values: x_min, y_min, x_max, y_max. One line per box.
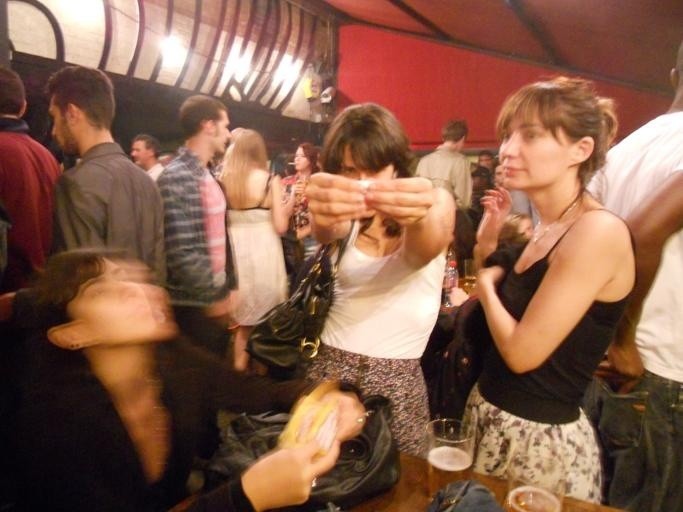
442, 247, 459, 308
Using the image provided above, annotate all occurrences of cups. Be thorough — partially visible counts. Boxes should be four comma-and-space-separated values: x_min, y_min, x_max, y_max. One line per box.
464, 259, 479, 289
422, 417, 477, 503
508, 458, 566, 510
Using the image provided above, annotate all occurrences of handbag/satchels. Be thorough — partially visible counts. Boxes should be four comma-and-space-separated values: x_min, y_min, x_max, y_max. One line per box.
202, 380, 403, 511
279, 217, 306, 275
245, 217, 357, 371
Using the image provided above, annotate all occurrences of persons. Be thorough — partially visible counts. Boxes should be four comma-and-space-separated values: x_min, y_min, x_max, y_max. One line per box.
0, 41, 682, 511
